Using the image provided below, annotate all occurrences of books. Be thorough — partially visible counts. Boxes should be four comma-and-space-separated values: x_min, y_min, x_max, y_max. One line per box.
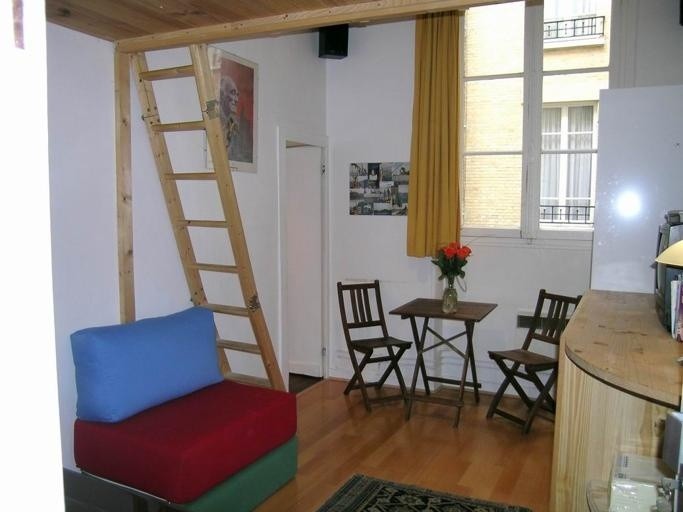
671, 271, 682, 342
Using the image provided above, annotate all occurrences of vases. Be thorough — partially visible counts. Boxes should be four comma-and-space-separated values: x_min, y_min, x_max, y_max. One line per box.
442, 282, 458, 314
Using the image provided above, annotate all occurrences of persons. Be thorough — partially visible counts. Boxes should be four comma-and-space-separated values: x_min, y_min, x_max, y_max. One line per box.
220, 74, 251, 162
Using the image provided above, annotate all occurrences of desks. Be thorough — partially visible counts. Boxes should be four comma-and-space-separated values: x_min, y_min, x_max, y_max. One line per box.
388, 297, 498, 431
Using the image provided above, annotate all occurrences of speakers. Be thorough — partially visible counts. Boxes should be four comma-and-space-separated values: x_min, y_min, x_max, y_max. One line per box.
318, 25, 349, 59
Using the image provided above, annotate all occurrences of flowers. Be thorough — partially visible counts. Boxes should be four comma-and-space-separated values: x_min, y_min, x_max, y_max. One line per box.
430, 240, 471, 306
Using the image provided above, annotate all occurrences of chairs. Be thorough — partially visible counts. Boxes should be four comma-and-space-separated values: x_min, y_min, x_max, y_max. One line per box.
72, 304, 299, 512
485, 288, 582, 435
336, 280, 412, 412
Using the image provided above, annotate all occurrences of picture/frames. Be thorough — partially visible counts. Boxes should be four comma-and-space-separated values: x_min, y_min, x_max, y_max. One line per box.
200, 42, 258, 174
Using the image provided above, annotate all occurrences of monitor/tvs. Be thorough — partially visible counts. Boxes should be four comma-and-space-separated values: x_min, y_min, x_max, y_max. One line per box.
652, 224, 683, 333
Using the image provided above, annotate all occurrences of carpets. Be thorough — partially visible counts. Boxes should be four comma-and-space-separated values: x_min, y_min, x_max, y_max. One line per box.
313, 471, 534, 512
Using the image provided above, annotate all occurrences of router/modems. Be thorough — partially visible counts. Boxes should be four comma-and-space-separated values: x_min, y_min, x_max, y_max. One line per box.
665, 211, 683, 226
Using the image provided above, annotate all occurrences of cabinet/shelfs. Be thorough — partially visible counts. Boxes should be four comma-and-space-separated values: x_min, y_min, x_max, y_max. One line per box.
547, 289, 683, 512
